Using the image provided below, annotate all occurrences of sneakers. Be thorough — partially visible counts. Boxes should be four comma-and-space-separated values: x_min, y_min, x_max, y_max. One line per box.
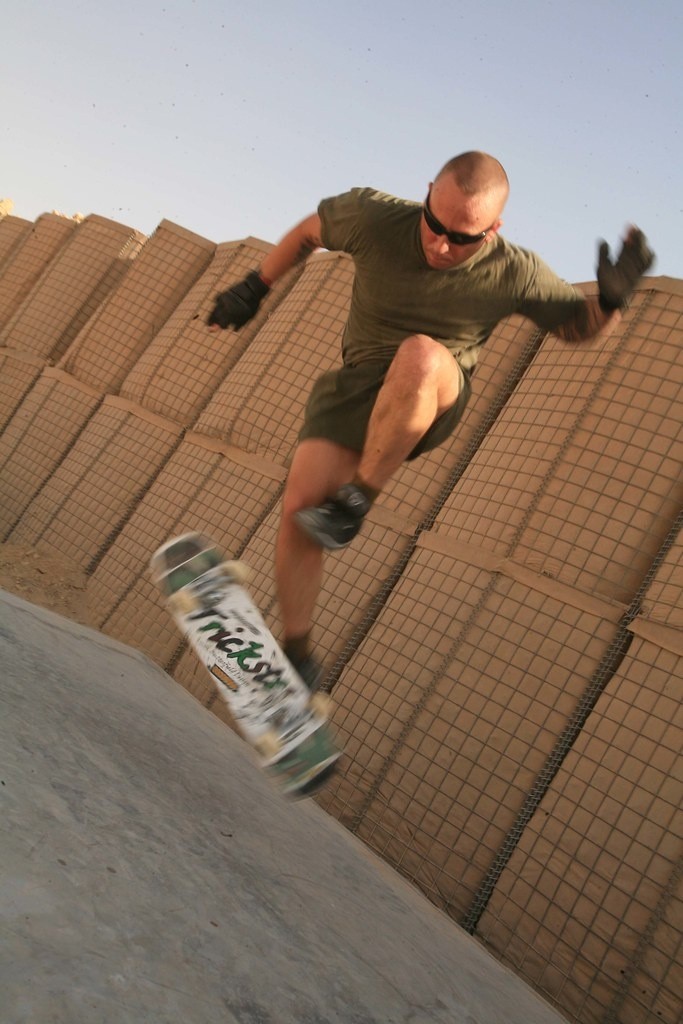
295, 483, 371, 550
291, 658, 324, 691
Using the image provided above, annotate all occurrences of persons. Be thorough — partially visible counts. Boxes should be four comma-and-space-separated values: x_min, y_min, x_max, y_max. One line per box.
207, 151, 656, 695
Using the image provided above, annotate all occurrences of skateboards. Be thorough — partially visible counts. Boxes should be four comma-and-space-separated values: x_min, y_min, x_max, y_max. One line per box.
147, 530, 349, 802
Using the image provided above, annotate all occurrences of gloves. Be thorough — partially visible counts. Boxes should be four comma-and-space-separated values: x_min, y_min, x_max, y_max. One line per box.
208, 271, 270, 331
597, 225, 654, 315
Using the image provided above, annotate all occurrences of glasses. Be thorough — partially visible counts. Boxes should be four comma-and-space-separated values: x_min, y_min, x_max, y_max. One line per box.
424, 191, 493, 245
